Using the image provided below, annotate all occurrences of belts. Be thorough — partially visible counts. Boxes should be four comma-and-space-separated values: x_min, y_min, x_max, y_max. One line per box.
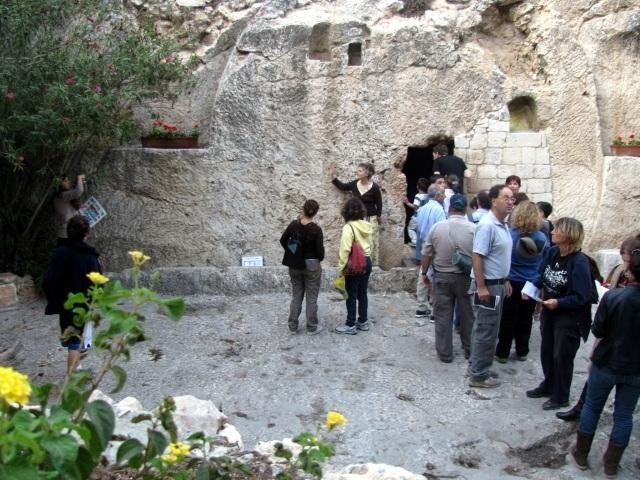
485, 279, 503, 285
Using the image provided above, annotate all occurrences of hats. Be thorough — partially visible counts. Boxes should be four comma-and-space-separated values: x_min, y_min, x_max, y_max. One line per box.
334, 276, 348, 299
450, 193, 467, 210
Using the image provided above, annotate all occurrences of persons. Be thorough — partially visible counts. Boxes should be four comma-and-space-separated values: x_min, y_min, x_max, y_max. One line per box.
519, 216, 606, 412
280, 199, 324, 336
338, 196, 374, 335
42, 216, 104, 379
555, 233, 640, 480
401, 140, 554, 388
328, 162, 383, 273
48, 174, 86, 252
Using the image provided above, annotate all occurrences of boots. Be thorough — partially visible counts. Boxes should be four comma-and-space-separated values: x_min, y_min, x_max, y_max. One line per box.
569, 430, 593, 469
556, 381, 587, 421
603, 442, 625, 478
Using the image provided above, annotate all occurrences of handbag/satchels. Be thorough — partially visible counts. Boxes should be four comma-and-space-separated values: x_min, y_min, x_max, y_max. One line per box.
342, 242, 367, 275
452, 252, 472, 274
282, 240, 305, 271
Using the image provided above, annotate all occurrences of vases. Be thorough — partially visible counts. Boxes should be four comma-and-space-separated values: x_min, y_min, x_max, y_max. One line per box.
142, 138, 199, 148
611, 146, 640, 156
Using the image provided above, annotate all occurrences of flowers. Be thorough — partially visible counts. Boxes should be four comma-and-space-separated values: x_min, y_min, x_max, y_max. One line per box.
140, 120, 201, 138
609, 134, 640, 146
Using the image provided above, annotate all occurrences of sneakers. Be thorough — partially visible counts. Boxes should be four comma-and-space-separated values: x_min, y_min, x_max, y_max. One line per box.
335, 325, 357, 335
307, 325, 323, 336
354, 320, 369, 330
464, 353, 571, 409
416, 310, 432, 317
